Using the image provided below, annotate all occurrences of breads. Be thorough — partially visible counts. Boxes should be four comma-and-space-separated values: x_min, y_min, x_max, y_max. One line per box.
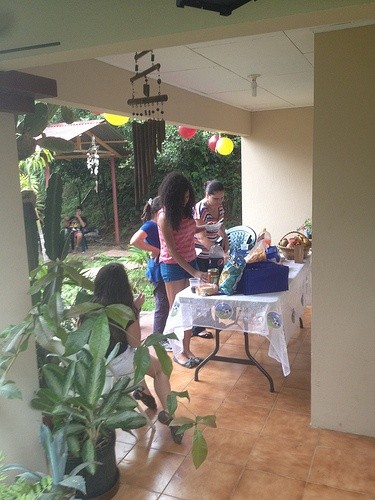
200, 287, 215, 296
246, 251, 264, 263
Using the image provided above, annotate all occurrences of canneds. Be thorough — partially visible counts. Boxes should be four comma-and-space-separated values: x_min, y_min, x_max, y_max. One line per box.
207, 268, 218, 287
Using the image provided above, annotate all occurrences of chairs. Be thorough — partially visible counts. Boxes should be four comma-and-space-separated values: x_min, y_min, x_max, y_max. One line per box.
216, 225, 256, 258
50, 336, 158, 433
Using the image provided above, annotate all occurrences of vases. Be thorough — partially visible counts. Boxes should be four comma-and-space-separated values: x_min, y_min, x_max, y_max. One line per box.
39, 414, 116, 499
307, 231, 312, 239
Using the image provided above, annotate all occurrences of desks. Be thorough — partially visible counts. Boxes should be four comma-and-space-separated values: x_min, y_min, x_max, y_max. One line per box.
163, 248, 312, 393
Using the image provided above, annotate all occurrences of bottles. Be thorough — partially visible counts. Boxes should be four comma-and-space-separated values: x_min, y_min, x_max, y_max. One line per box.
208, 243, 224, 273
208, 268, 219, 286
257, 228, 271, 249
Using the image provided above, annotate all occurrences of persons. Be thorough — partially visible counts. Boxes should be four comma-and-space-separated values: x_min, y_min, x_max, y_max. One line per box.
63, 207, 87, 254
76, 263, 184, 442
130, 196, 174, 352
192, 180, 228, 338
156, 173, 209, 368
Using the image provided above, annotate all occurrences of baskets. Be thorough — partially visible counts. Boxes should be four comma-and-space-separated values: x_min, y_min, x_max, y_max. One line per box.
277, 231, 311, 260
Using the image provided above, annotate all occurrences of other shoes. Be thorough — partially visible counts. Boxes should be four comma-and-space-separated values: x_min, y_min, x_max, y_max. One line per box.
183, 354, 204, 364
161, 343, 173, 351
173, 356, 195, 367
193, 332, 213, 338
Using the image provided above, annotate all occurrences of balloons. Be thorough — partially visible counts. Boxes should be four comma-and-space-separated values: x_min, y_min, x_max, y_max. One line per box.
208, 135, 220, 152
216, 138, 234, 155
103, 113, 129, 126
179, 126, 198, 138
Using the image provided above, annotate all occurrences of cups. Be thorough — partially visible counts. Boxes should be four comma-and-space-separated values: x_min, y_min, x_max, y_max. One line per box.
189, 277, 200, 293
294, 245, 304, 263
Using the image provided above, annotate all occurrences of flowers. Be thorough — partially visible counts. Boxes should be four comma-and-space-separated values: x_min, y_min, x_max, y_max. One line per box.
296, 218, 312, 240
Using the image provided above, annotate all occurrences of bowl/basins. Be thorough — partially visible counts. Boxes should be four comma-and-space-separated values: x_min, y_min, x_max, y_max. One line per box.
206, 223, 223, 231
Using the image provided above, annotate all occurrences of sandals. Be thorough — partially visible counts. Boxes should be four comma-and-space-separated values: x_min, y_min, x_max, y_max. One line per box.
158, 410, 182, 443
133, 389, 157, 410
